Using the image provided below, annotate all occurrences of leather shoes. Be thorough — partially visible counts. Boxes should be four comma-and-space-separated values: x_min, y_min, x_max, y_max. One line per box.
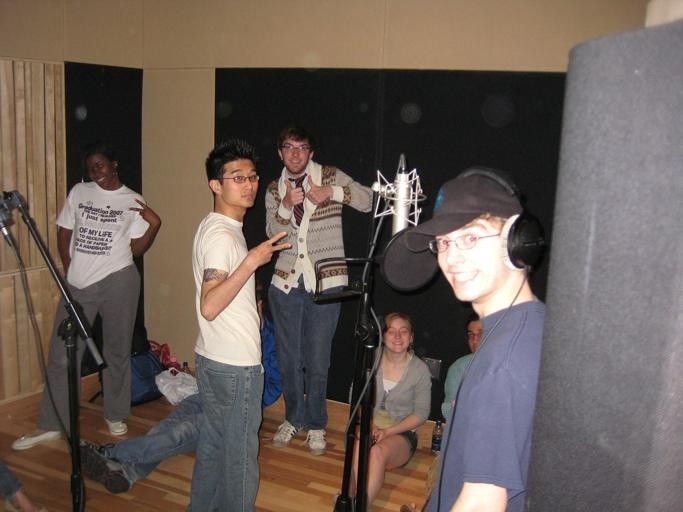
104, 415, 129, 437
12, 429, 62, 449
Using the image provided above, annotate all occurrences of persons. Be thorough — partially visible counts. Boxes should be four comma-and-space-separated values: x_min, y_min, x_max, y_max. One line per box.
12, 144, 164, 451
262, 128, 371, 457
0, 457, 33, 512
343, 308, 432, 512
191, 138, 292, 512
68, 285, 283, 496
404, 166, 545, 512
441, 317, 490, 425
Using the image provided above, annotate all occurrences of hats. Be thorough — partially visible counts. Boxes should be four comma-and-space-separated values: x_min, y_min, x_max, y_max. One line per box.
405, 175, 524, 253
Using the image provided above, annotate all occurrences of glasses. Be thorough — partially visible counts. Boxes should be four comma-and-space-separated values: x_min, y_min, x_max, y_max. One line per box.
279, 141, 313, 152
219, 175, 260, 183
429, 233, 502, 254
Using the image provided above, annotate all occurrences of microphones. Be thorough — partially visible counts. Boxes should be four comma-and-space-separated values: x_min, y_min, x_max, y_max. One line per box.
0, 188, 18, 244
379, 156, 438, 293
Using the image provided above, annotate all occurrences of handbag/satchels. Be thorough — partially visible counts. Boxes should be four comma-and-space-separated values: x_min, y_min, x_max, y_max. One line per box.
106, 341, 167, 409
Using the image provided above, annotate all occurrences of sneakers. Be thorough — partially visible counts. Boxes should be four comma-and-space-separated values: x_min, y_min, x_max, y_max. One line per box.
307, 428, 326, 456
68, 436, 135, 496
273, 418, 301, 447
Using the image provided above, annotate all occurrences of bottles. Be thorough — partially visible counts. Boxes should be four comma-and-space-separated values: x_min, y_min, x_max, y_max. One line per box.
182, 362, 191, 375
431, 419, 443, 456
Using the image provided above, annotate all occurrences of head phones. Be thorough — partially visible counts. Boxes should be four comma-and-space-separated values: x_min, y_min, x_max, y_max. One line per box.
455, 166, 547, 269
